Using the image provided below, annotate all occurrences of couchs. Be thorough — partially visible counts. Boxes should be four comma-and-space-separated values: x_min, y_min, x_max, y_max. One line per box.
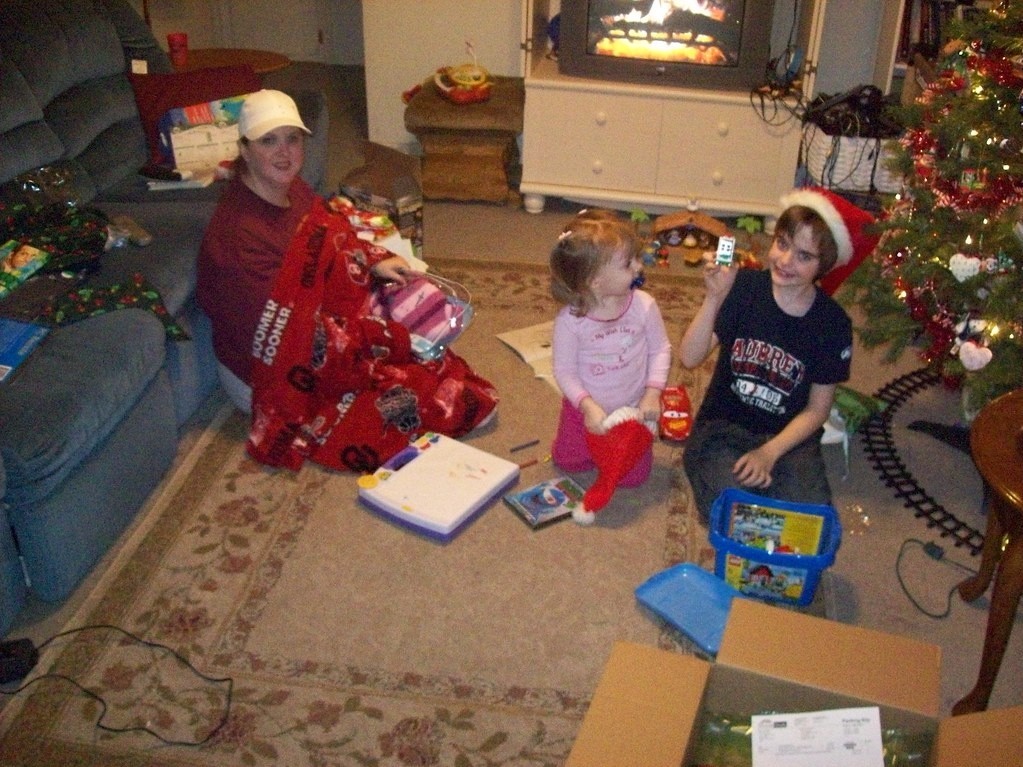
0, 0, 331, 637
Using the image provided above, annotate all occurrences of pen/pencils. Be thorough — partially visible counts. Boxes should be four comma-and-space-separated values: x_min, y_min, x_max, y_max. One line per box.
509, 439, 540, 452
519, 459, 538, 468
543, 454, 552, 463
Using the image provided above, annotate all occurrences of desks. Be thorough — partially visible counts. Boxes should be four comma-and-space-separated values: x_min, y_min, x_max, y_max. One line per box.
951, 386, 1023, 718
164, 50, 290, 75
409, 75, 521, 209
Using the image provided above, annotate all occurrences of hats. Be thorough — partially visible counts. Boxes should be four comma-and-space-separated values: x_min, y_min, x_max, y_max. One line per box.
780, 183, 886, 298
572, 406, 659, 527
238, 88, 312, 142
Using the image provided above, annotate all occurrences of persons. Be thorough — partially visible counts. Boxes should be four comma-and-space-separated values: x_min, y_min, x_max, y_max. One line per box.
0, 242, 40, 289
546, 208, 674, 488
679, 186, 885, 523
197, 83, 411, 418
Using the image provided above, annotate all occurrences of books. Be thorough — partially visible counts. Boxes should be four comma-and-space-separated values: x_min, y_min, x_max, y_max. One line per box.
0, 239, 50, 298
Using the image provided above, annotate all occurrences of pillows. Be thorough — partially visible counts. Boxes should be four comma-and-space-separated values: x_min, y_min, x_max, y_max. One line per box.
129, 65, 261, 165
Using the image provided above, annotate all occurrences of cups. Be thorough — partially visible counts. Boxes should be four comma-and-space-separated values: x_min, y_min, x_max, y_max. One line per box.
166, 33, 188, 65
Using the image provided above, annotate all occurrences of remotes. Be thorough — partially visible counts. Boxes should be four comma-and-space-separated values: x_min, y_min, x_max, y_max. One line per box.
111, 215, 151, 246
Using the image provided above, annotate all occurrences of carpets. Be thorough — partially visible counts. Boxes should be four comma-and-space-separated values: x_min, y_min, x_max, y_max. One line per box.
1, 261, 836, 767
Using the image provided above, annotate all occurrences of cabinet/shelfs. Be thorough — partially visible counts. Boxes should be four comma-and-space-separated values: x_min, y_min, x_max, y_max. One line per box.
520, 52, 807, 237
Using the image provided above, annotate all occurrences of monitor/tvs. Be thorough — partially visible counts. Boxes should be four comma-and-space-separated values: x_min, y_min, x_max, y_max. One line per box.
558, 0, 776, 91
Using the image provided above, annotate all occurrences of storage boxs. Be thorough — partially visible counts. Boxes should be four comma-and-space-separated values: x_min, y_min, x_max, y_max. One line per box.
564, 598, 1023, 766
340, 139, 423, 257
802, 121, 906, 194
710, 488, 844, 605
379, 269, 473, 362
